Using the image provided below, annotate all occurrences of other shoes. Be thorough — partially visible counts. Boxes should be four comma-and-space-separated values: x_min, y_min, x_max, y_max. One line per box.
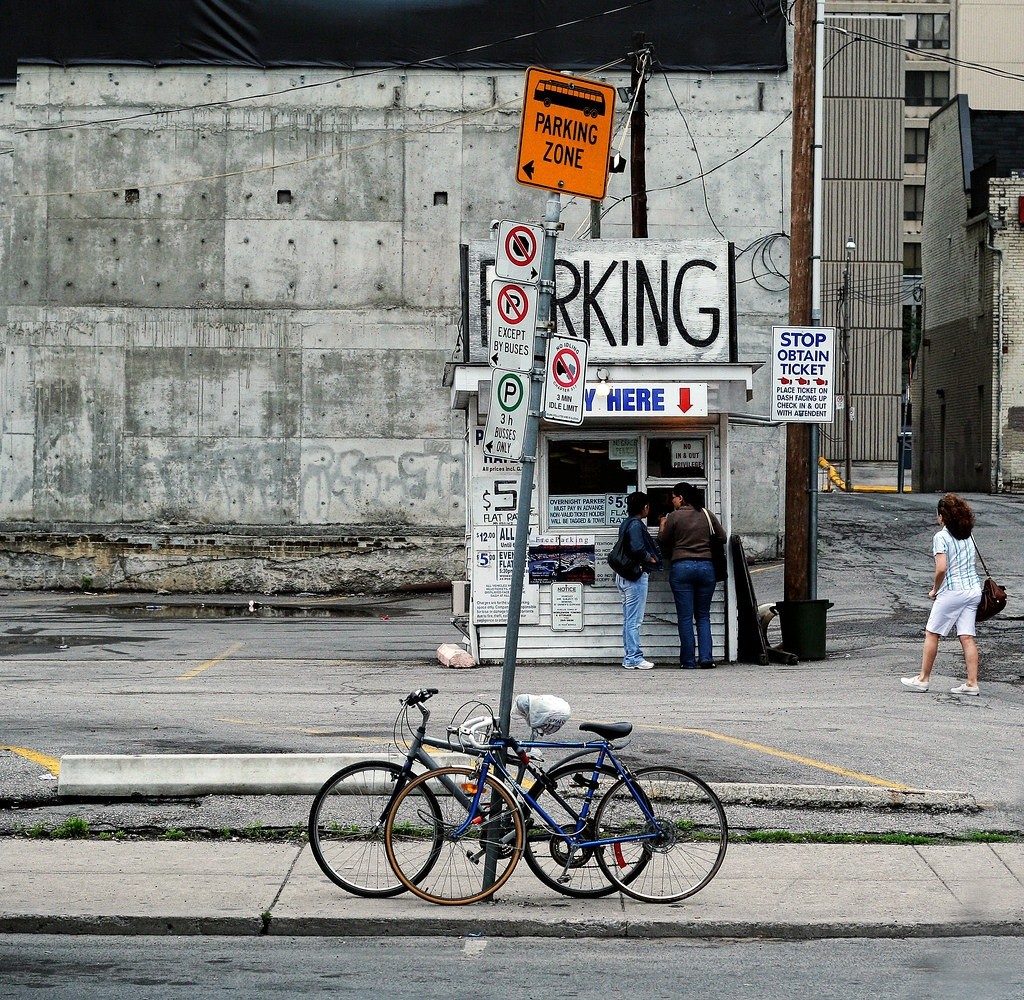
681, 663, 695, 669
700, 661, 717, 669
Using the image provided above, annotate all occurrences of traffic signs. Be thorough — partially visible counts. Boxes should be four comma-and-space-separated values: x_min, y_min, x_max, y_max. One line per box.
771, 326, 836, 424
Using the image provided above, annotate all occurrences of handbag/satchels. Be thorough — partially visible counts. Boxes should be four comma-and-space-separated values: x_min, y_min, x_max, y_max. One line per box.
703, 508, 728, 582
976, 578, 1007, 622
608, 520, 647, 582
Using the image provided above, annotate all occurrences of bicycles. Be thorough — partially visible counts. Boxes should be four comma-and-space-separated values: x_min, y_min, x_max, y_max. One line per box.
308, 687, 657, 899
384, 700, 728, 906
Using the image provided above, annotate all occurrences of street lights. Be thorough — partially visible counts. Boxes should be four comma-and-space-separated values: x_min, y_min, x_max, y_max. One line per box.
843, 235, 857, 490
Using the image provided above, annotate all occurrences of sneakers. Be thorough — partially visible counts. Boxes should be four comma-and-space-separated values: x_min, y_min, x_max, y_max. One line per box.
950, 683, 980, 695
622, 659, 654, 669
901, 676, 929, 691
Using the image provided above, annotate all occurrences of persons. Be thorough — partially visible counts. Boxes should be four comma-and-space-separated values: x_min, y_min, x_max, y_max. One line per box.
900, 491, 982, 696
657, 482, 728, 670
615, 492, 660, 670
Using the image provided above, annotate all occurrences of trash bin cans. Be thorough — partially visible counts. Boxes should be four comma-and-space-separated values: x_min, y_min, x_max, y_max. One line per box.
775, 598, 835, 663
